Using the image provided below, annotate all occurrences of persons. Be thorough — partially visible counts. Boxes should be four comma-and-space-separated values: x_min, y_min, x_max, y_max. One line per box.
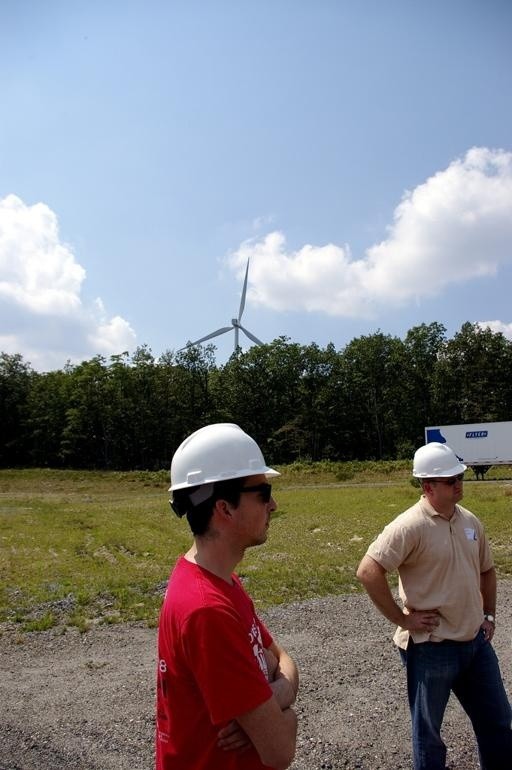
152, 421, 303, 769
356, 440, 510, 770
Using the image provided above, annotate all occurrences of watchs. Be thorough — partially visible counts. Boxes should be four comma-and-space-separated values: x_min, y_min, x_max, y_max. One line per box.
484, 612, 496, 626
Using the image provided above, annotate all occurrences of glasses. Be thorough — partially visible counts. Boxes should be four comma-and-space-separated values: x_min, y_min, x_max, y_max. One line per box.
238, 483, 272, 504
429, 473, 464, 486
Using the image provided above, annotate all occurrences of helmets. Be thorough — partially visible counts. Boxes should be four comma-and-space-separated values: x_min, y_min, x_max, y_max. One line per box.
168, 422, 283, 493
413, 441, 467, 478
